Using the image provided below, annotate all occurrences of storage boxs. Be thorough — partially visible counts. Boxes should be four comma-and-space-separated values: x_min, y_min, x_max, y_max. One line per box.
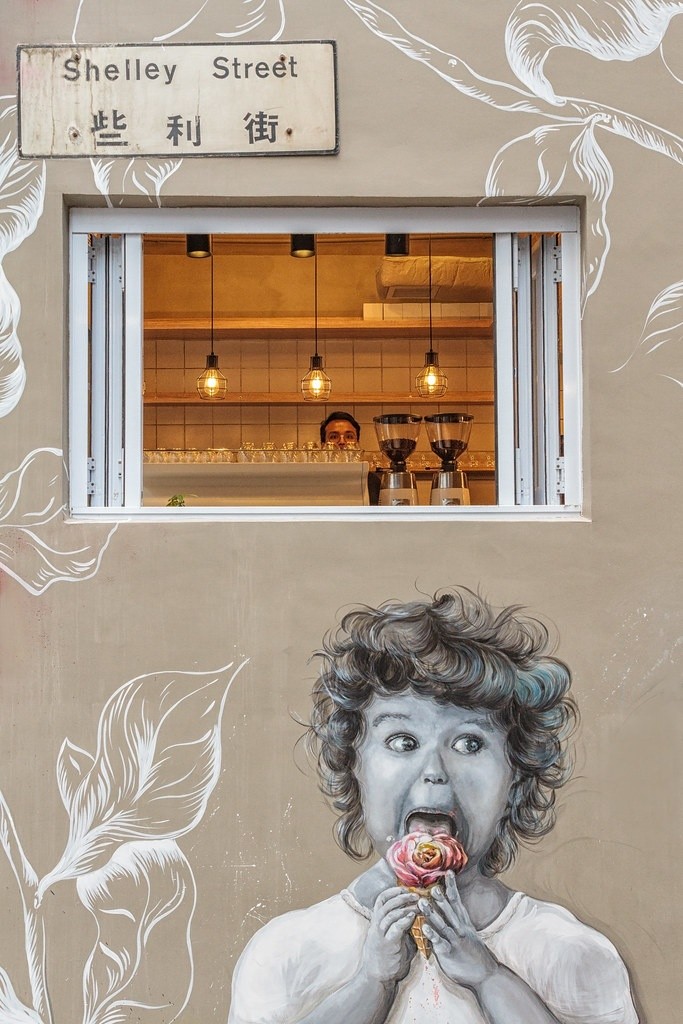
363, 303, 493, 320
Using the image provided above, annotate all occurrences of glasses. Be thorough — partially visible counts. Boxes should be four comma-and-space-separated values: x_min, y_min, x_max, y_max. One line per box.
324, 431, 358, 444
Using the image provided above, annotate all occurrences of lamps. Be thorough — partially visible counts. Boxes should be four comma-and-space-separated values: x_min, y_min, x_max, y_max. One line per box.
385, 234, 410, 257
186, 235, 211, 259
415, 233, 448, 399
196, 234, 227, 401
290, 236, 315, 257
300, 234, 332, 401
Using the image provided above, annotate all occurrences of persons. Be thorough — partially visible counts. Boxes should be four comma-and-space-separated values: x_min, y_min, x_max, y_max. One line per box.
320, 411, 381, 506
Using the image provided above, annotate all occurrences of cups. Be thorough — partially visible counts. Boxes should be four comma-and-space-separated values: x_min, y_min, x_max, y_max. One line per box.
143, 442, 363, 463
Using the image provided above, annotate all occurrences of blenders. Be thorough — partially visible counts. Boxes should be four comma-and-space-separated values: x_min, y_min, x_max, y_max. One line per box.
372, 414, 423, 506
424, 412, 474, 505
